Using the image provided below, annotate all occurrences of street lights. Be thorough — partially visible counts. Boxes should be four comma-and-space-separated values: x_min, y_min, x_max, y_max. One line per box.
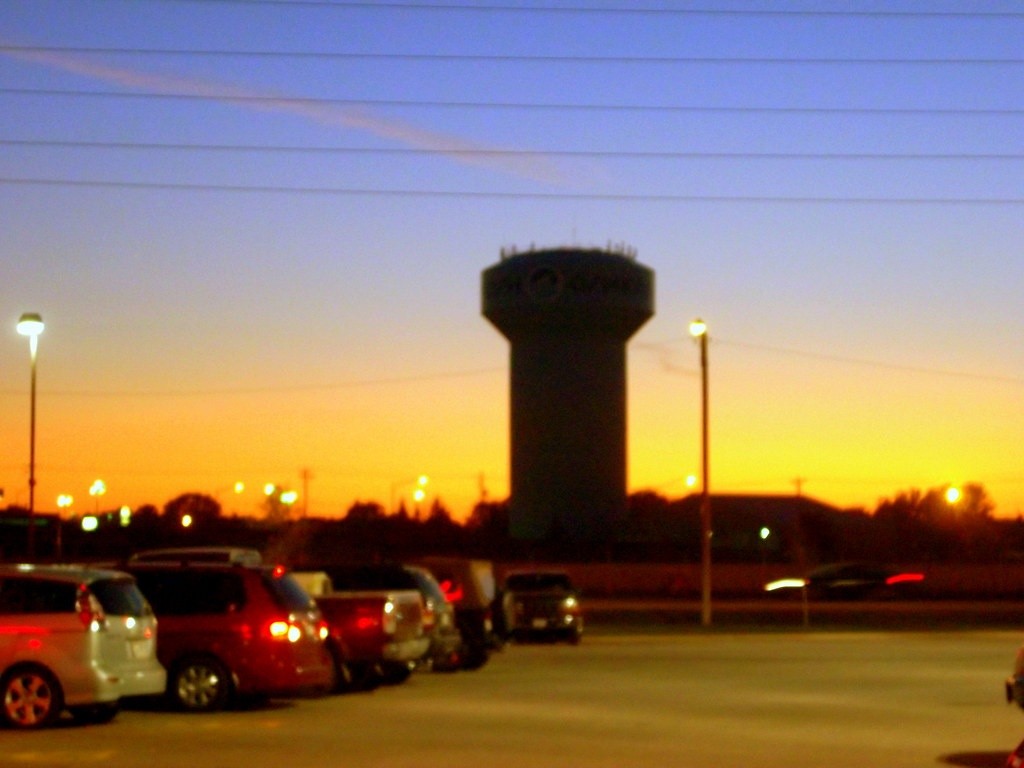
686, 317, 713, 627
15, 311, 44, 563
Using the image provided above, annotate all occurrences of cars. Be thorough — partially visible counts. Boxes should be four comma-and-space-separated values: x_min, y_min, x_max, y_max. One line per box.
762, 556, 932, 604
501, 569, 585, 645
0, 539, 500, 734
